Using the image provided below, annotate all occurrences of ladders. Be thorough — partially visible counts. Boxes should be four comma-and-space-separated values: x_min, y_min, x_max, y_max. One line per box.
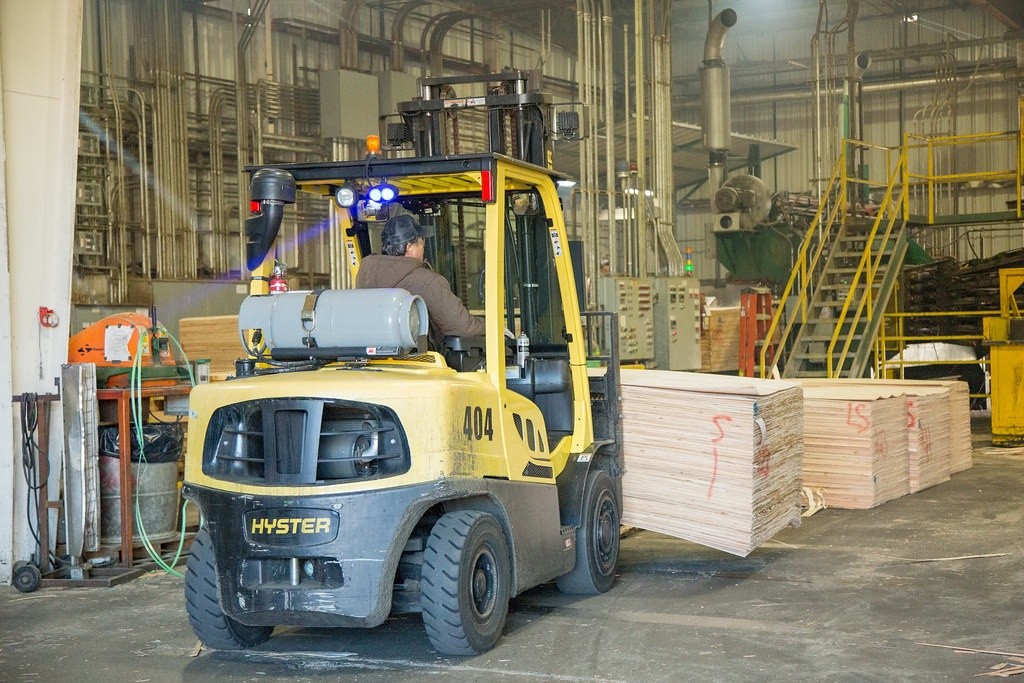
737, 285, 775, 379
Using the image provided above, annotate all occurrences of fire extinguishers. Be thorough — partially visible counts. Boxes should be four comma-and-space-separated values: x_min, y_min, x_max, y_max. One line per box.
269, 258, 288, 294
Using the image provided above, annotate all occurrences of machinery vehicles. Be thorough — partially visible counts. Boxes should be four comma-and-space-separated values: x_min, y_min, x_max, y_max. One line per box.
180, 7, 738, 658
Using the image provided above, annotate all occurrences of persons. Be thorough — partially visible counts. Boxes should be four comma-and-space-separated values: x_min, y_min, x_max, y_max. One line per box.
356, 213, 515, 354
